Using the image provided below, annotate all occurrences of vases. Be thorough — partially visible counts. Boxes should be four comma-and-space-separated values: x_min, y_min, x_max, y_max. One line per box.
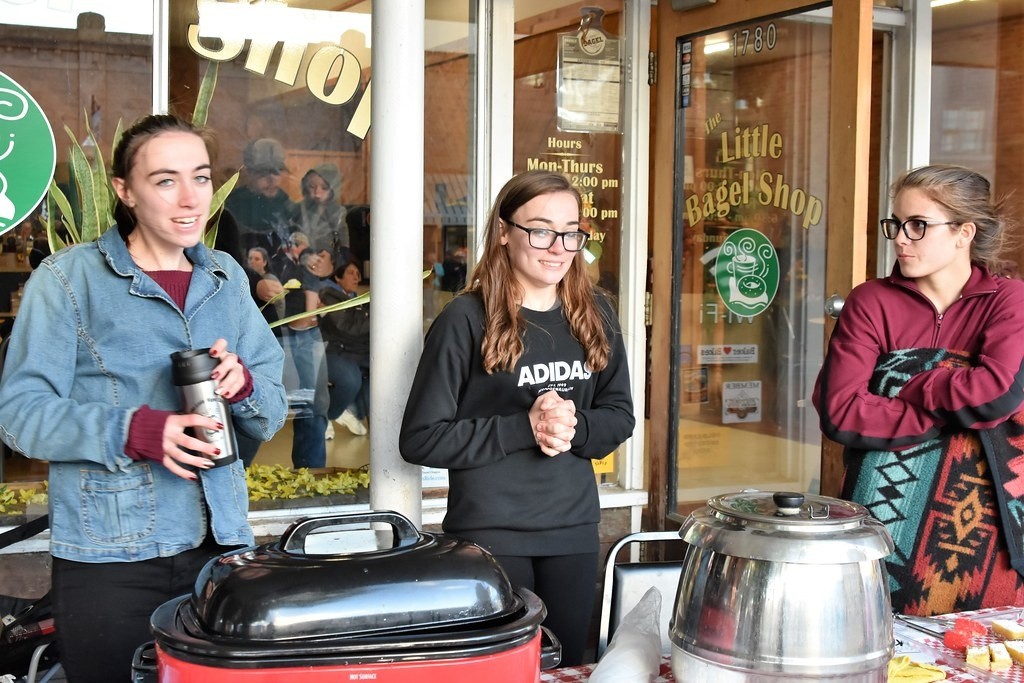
0, 482, 48, 526
246, 466, 370, 512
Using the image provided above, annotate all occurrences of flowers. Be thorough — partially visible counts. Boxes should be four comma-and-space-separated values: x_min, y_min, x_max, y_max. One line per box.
0, 478, 47, 514
244, 462, 370, 502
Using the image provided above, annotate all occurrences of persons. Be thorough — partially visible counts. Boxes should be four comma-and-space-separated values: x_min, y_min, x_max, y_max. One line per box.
399, 170, 637, 667
0, 114, 289, 683
210, 138, 370, 468
811, 164, 1024, 616
247, 247, 286, 320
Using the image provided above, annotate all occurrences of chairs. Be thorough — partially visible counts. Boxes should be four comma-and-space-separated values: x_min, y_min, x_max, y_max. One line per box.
597, 530, 688, 664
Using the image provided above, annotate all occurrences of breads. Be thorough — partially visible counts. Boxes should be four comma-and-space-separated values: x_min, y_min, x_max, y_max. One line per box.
989, 643, 1012, 667
991, 620, 1024, 640
967, 646, 989, 670
1004, 641, 1024, 666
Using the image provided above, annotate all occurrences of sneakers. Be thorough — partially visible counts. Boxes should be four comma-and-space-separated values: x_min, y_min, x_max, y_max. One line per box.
324, 421, 336, 442
336, 407, 370, 436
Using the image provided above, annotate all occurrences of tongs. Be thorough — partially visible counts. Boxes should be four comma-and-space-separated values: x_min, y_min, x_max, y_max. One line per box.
892, 612, 987, 652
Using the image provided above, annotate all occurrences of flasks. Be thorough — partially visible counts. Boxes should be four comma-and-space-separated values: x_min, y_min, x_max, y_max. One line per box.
170, 348, 238, 469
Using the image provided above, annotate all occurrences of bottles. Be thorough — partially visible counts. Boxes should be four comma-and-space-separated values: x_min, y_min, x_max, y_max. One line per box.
15, 236, 24, 262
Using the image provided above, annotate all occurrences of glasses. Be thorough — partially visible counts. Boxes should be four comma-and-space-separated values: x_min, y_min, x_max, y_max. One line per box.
501, 218, 590, 255
880, 215, 957, 241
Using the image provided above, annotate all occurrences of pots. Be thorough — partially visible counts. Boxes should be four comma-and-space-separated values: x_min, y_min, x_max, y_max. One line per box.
130, 508, 563, 683
669, 491, 895, 683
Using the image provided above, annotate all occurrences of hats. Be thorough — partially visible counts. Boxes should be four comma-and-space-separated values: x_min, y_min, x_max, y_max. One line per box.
242, 136, 295, 177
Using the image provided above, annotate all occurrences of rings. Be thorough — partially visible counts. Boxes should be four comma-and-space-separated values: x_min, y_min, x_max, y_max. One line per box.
311, 265, 316, 270
